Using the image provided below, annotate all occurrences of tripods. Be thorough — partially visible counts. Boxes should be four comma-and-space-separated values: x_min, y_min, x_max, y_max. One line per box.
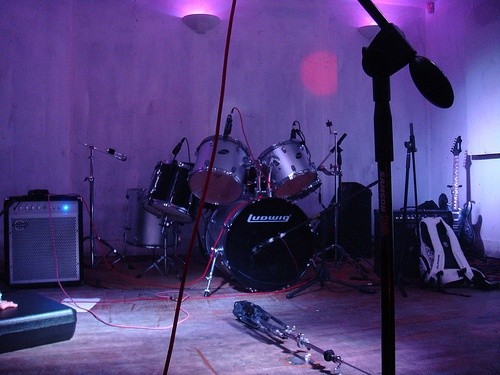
136, 217, 182, 280
79, 144, 134, 270
263, 120, 377, 300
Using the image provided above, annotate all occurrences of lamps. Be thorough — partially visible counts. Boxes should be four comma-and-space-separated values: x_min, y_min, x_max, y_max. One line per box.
358, 24, 382, 41
181, 13, 219, 35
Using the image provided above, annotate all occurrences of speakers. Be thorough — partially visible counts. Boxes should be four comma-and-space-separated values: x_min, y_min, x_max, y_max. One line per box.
4, 193, 85, 287
373, 208, 453, 287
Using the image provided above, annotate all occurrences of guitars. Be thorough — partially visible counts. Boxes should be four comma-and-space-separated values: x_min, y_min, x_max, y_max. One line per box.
445, 135, 475, 254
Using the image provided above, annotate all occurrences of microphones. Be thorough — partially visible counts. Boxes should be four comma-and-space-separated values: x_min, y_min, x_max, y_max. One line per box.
172, 138, 185, 155
107, 147, 127, 161
252, 244, 261, 255
291, 122, 297, 140
225, 109, 234, 135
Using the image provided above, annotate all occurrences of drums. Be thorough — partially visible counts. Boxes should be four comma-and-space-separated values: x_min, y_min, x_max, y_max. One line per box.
205, 194, 314, 291
187, 135, 251, 205
143, 160, 199, 223
123, 188, 181, 249
258, 139, 317, 201
286, 177, 322, 201
